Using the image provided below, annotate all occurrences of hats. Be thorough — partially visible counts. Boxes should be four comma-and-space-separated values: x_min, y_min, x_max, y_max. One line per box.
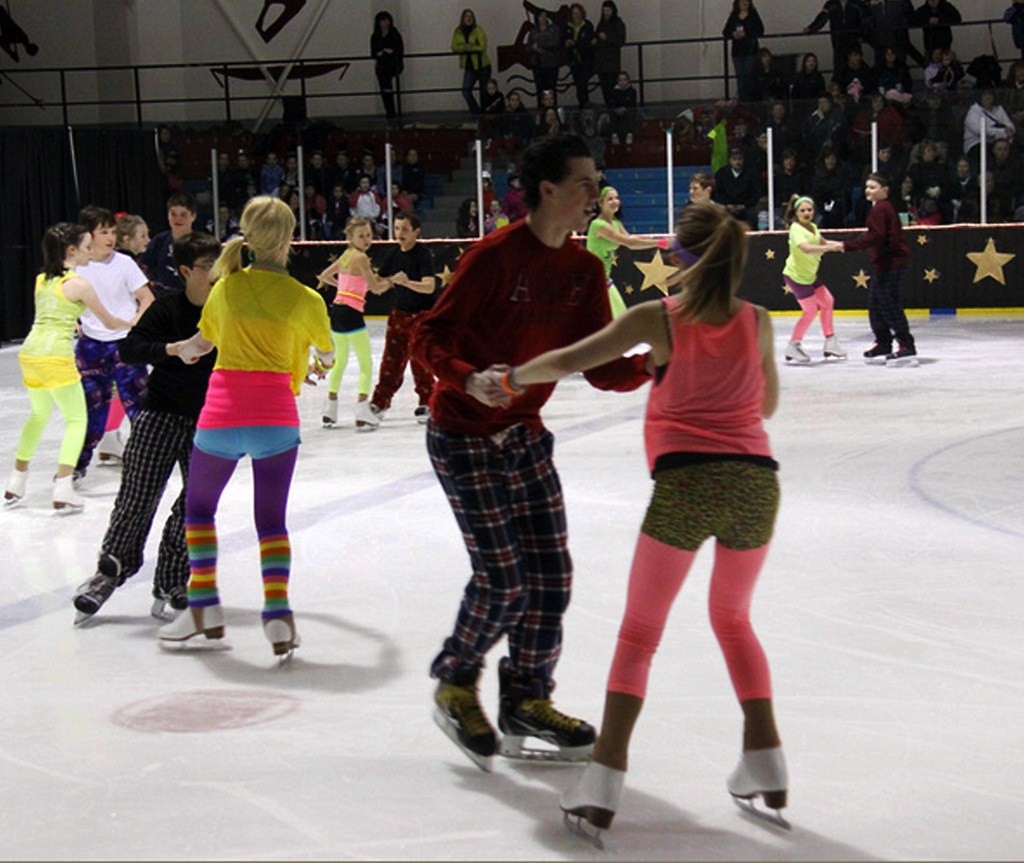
482, 170, 491, 179
728, 147, 744, 158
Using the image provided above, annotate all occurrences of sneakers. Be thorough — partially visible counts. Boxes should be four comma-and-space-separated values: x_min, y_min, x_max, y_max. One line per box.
355, 400, 380, 432
431, 681, 502, 773
886, 344, 919, 367
157, 604, 231, 651
262, 609, 299, 665
785, 340, 810, 365
98, 430, 126, 468
323, 397, 339, 428
824, 336, 847, 362
151, 582, 189, 621
4, 468, 29, 506
73, 553, 127, 625
560, 761, 625, 850
52, 475, 86, 517
727, 744, 790, 829
498, 691, 597, 763
863, 344, 892, 364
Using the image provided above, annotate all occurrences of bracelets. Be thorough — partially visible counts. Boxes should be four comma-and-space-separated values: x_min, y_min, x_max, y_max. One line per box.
386, 279, 395, 290
501, 368, 528, 397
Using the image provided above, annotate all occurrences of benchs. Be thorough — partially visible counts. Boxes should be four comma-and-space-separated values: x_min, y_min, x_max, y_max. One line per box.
171, 117, 743, 234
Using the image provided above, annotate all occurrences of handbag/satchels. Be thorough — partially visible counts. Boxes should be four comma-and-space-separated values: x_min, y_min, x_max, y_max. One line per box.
983, 111, 1007, 128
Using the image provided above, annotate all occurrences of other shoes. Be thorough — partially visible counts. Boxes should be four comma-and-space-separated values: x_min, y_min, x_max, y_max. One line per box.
414, 405, 430, 424
613, 133, 621, 145
626, 133, 633, 145
369, 403, 385, 421
53, 467, 87, 489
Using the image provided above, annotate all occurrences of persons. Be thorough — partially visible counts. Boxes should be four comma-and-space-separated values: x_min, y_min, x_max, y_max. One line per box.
782, 197, 848, 361
72, 194, 337, 653
0, 194, 219, 509
839, 168, 917, 361
359, 132, 663, 756
662, 172, 726, 254
139, 0, 1024, 240
587, 186, 660, 320
492, 204, 788, 836
319, 214, 434, 426
